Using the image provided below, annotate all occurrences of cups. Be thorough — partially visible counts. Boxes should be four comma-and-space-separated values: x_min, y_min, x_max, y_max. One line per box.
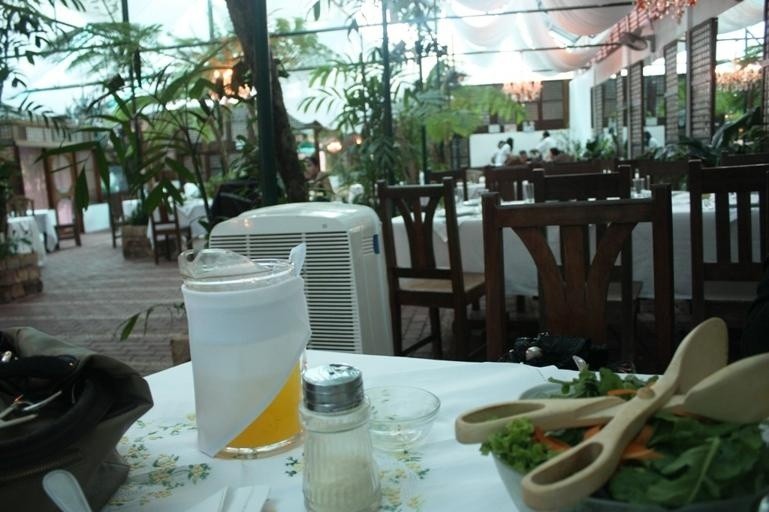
526, 184, 535, 203
632, 178, 644, 197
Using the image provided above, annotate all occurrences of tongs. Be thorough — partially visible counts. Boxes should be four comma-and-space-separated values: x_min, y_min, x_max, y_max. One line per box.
455, 318, 768, 511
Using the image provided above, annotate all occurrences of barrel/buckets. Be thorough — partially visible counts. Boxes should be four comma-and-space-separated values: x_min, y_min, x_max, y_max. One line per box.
178, 249, 302, 460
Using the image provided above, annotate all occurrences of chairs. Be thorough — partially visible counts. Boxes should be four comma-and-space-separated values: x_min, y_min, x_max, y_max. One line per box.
376, 178, 484, 362
484, 164, 541, 202
55, 199, 82, 248
719, 150, 768, 166
148, 197, 193, 265
533, 163, 643, 363
107, 194, 129, 248
481, 184, 676, 375
614, 156, 647, 179
676, 159, 769, 334
424, 167, 468, 203
7, 196, 34, 217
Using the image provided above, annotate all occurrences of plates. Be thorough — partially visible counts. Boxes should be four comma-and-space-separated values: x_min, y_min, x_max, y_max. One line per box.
729, 193, 760, 207
436, 205, 474, 218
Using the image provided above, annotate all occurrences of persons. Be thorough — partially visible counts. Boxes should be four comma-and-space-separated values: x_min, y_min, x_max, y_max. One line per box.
643, 131, 662, 158
303, 157, 333, 201
491, 131, 565, 167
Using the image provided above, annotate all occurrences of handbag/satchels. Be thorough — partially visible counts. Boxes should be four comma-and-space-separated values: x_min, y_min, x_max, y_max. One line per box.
0, 326, 154, 512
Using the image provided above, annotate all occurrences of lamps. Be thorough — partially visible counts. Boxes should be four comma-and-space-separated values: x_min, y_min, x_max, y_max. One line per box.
500, 54, 543, 106
715, 40, 764, 96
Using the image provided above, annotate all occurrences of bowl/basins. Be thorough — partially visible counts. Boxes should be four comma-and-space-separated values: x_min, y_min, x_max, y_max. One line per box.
493, 381, 767, 511
351, 384, 441, 450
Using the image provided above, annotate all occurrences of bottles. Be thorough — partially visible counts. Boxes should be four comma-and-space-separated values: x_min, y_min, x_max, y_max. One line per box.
298, 363, 381, 512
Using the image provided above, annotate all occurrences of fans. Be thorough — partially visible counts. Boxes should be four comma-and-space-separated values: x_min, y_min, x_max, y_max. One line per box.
618, 27, 655, 52
